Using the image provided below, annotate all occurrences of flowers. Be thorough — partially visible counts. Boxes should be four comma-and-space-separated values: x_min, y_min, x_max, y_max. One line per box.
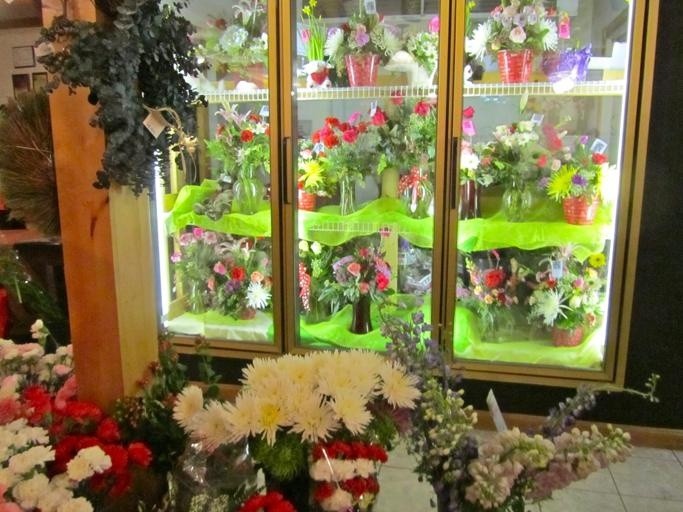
539, 134, 606, 187
0, 316, 633, 506
172, 227, 275, 311
297, 114, 370, 194
212, 101, 272, 147
476, 116, 562, 189
463, 0, 571, 53
398, 244, 532, 306
367, 90, 481, 171
197, 1, 270, 66
528, 243, 609, 331
298, 0, 441, 65
295, 227, 394, 313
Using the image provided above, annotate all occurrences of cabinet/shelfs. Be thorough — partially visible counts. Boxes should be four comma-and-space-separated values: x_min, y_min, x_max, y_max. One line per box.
148, 2, 665, 392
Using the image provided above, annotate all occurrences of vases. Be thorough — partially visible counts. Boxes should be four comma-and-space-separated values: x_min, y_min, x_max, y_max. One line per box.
232, 61, 268, 91
335, 173, 358, 215
498, 173, 537, 222
341, 50, 382, 85
297, 183, 320, 212
495, 48, 534, 83
401, 155, 437, 219
561, 195, 601, 224
347, 294, 374, 335
387, 50, 438, 85
229, 161, 266, 215
299, 298, 334, 325
378, 163, 400, 201
547, 319, 584, 349
232, 298, 256, 320
456, 180, 483, 221
472, 303, 506, 343
296, 60, 339, 89
